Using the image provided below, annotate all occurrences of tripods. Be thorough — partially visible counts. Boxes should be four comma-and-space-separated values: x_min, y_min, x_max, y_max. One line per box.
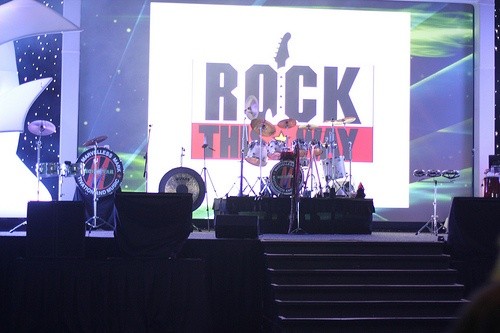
223, 114, 356, 198
290, 198, 306, 235
83, 142, 115, 234
414, 181, 448, 236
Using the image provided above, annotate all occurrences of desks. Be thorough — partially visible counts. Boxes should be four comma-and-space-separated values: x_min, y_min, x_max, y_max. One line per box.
212, 198, 376, 236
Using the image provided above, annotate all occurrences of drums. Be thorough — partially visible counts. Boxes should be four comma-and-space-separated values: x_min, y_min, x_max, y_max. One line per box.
35, 162, 60, 178
413, 169, 461, 179
56, 163, 86, 178
245, 141, 268, 167
269, 161, 304, 197
267, 140, 289, 160
292, 138, 308, 157
74, 147, 123, 197
310, 141, 324, 155
321, 155, 346, 180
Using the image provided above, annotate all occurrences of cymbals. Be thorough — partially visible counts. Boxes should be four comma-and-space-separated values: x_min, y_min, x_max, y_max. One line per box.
28, 119, 56, 136
299, 123, 317, 130
321, 142, 338, 147
321, 120, 343, 126
250, 118, 276, 137
335, 117, 356, 124
277, 119, 297, 129
83, 136, 108, 145
246, 94, 259, 120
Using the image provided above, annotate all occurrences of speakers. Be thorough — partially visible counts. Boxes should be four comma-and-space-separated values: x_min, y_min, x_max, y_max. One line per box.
27, 200, 85, 237
448, 198, 500, 242
214, 214, 260, 239
